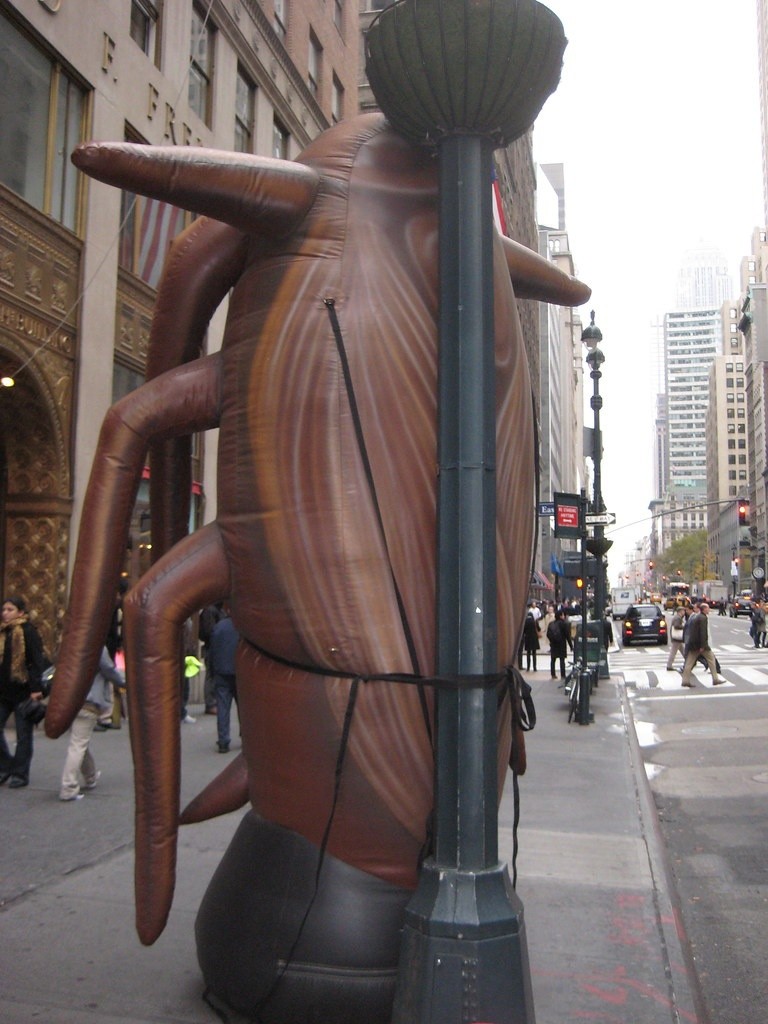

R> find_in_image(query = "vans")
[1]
[741,588,755,600]
[650,592,662,604]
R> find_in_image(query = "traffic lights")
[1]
[677,569,681,575]
[581,538,595,555]
[662,575,666,580]
[649,559,653,570]
[576,578,583,587]
[739,501,750,526]
[602,539,613,556]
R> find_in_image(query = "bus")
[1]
[663,581,690,597]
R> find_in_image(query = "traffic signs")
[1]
[585,513,616,526]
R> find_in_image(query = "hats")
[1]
[677,606,684,611]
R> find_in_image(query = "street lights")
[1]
[579,308,606,621]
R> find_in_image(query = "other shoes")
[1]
[85,770,101,788]
[183,716,197,724]
[682,683,695,687]
[713,680,726,685]
[60,793,84,801]
[10,776,26,787]
[205,706,217,714]
[667,668,676,670]
[0,770,10,784]
[219,745,229,752]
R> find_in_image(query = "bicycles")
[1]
[563,655,583,724]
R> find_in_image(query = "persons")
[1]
[528,596,594,637]
[53,629,125,800]
[201,600,225,713]
[0,596,43,788]
[639,598,642,603]
[547,611,574,678]
[210,604,237,752]
[682,595,691,606]
[672,598,679,616]
[603,615,613,650]
[524,612,541,671]
[750,601,768,648]
[181,618,196,722]
[681,603,727,687]
[98,580,129,729]
[718,597,727,616]
[666,603,721,673]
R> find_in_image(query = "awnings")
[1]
[530,572,553,590]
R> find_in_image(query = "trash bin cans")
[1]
[576,622,611,679]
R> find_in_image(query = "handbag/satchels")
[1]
[20,699,47,724]
[672,625,683,642]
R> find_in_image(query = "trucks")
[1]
[697,579,729,608]
[612,588,639,621]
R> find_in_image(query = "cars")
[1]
[646,591,653,599]
[729,597,754,618]
[622,604,669,648]
[663,596,689,611]
